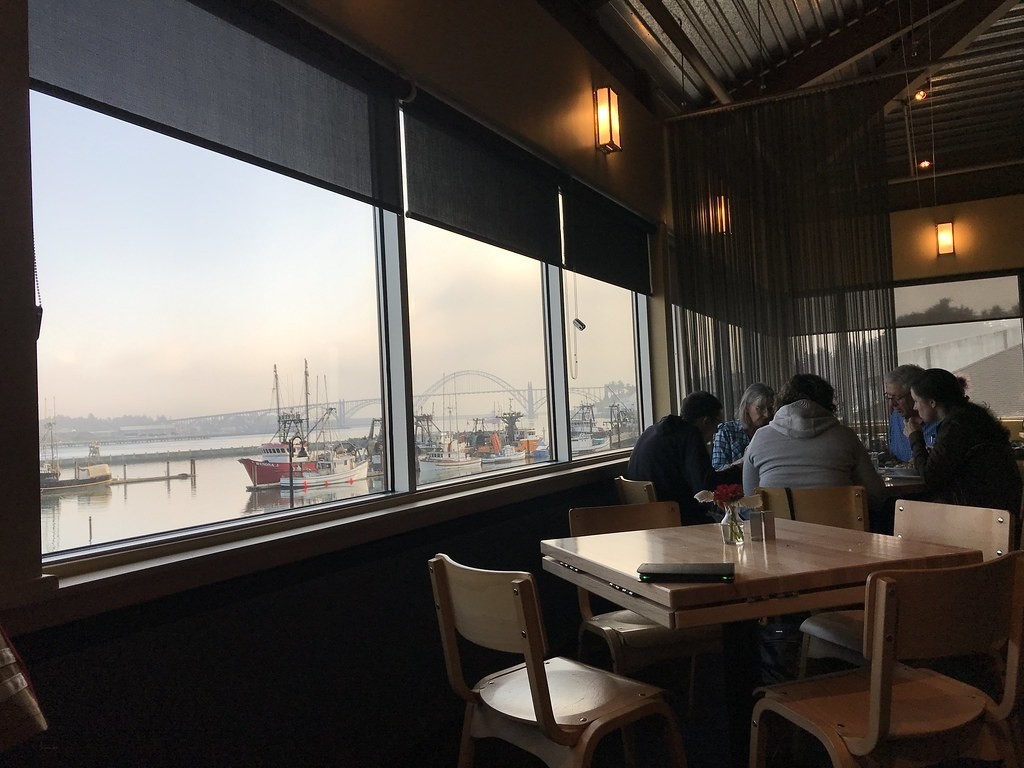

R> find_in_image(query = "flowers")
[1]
[694,483,744,545]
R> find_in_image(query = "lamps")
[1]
[917,159,931,169]
[914,90,927,101]
[591,83,623,156]
[935,222,955,255]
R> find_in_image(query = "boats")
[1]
[38,394,119,495]
[237,356,638,494]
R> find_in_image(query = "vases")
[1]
[720,506,744,546]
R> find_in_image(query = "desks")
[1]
[540,516,983,768]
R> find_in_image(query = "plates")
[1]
[884,475,919,478]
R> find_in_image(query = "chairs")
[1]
[569,501,723,768]
[753,486,870,531]
[1001,415,1024,520]
[427,552,688,768]
[613,475,657,504]
[749,550,1024,768]
[790,499,1024,768]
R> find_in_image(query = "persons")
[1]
[712,382,777,489]
[743,373,883,510]
[883,365,940,466]
[628,391,725,527]
[903,369,1024,550]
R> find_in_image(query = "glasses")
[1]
[751,404,777,416]
[883,392,910,400]
[702,417,725,429]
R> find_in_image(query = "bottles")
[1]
[871,452,878,471]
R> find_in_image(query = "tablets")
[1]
[637,562,736,584]
